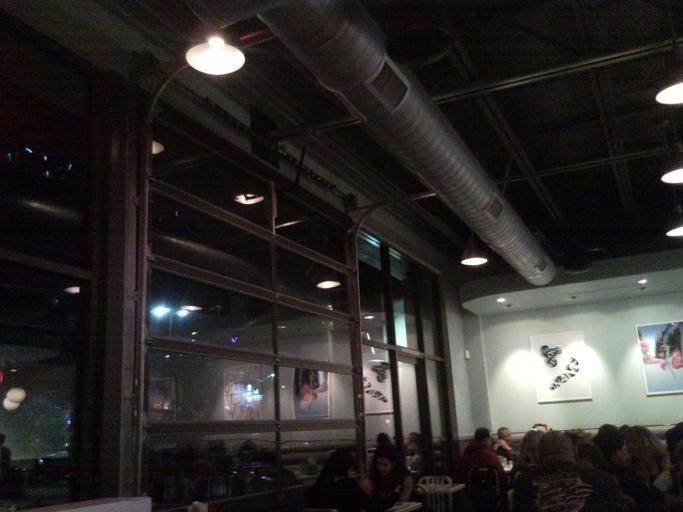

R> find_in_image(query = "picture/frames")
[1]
[529,331,593,405]
[358,359,394,416]
[221,361,263,422]
[634,319,683,398]
[291,356,332,421]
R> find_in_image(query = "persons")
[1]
[141,437,306,510]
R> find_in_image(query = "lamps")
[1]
[183,22,246,78]
[459,223,491,267]
